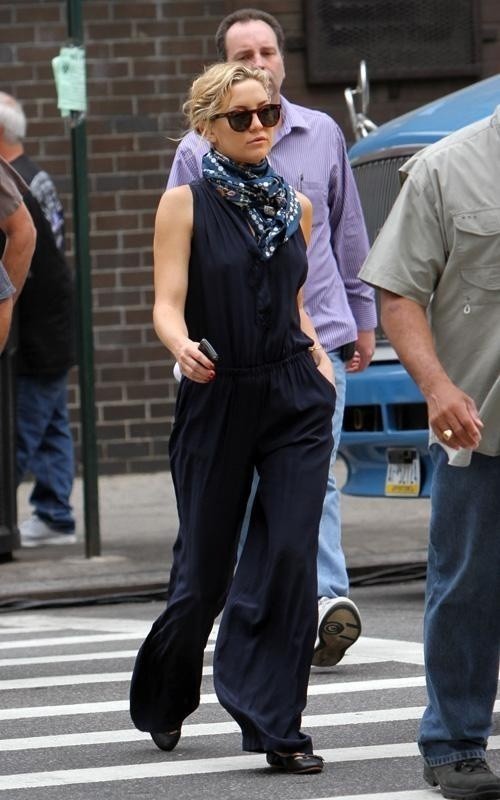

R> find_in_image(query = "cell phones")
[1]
[197,338,220,363]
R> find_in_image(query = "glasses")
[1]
[206,104,281,132]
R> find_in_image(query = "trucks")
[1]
[336,60,500,498]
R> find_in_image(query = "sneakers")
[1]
[17,515,77,548]
[311,596,362,667]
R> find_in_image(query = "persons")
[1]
[165,8,377,665]
[131,60,338,775]
[357,102,500,798]
[0,91,80,549]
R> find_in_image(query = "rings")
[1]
[442,429,453,441]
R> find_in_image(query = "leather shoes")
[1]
[0,552,12,563]
[149,731,181,751]
[422,758,499,800]
[266,750,325,774]
